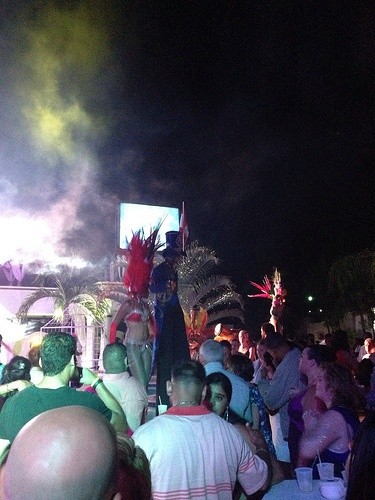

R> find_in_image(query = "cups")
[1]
[158,405,168,415]
[295,467,312,492]
[316,463,334,480]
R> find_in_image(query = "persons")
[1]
[109,279,156,398]
[150,247,194,406]
[0,321,375,499]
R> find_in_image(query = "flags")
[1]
[178,212,191,244]
